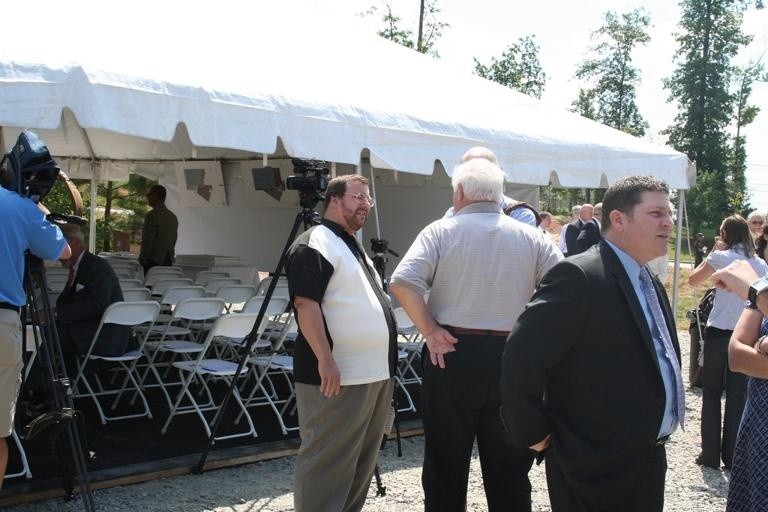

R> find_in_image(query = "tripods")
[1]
[191,208,388,497]
[22,251,97,512]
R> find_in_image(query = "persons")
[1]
[499,173,687,511]
[25,222,132,400]
[389,157,562,511]
[439,145,535,225]
[283,172,398,511]
[137,184,177,276]
[0,167,71,492]
[688,212,767,511]
[539,204,604,257]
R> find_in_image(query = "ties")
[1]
[640,268,685,432]
[67,268,74,284]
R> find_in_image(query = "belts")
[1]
[448,326,509,337]
[655,435,671,446]
[0,302,20,312]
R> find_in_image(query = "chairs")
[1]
[0,324,42,480]
[389,295,429,415]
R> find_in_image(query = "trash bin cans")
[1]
[687,309,727,389]
[113,227,130,251]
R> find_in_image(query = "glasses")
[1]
[345,192,376,207]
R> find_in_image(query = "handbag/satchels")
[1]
[693,288,715,320]
